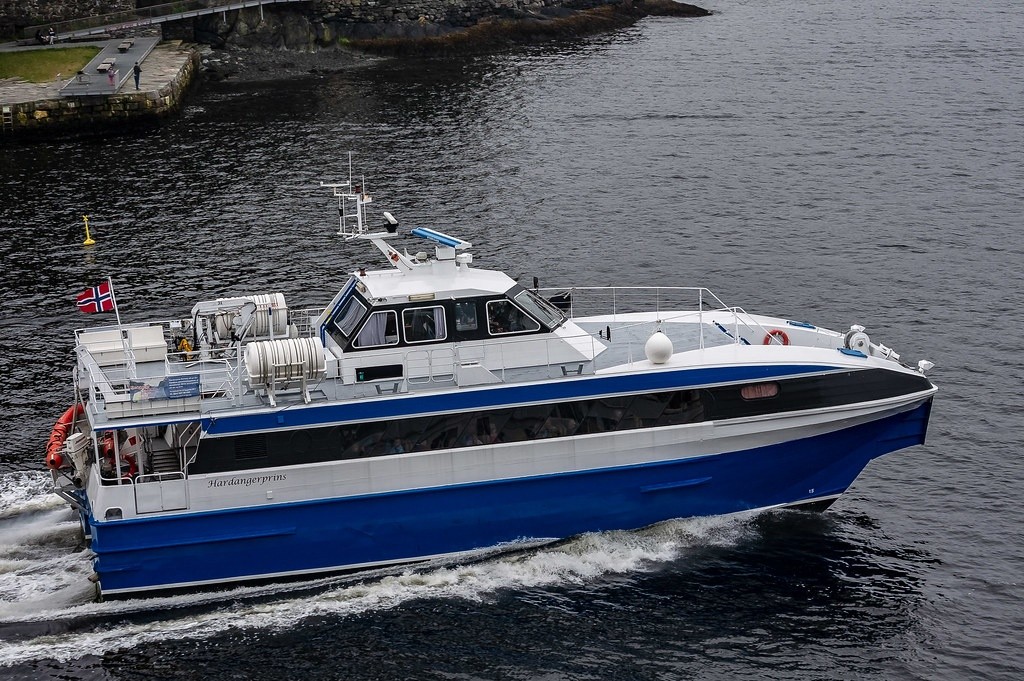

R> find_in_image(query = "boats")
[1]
[45,151,938,603]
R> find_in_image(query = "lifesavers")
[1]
[764,330,788,345]
[108,454,135,480]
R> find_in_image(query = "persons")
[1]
[489,301,512,334]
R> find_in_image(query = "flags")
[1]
[72,280,115,314]
